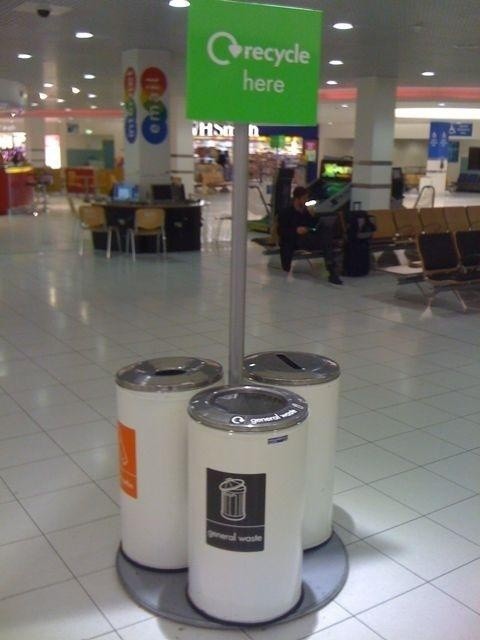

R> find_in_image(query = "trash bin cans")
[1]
[186,385,309,627]
[243,353,341,553]
[114,358,224,573]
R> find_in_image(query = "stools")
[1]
[26,181,49,216]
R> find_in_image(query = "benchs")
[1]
[206,173,227,186]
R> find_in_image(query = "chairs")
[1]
[367,210,415,240]
[420,208,449,232]
[444,207,472,232]
[467,206,480,230]
[416,232,480,313]
[79,206,121,258]
[127,208,167,261]
[393,208,421,238]
[454,231,480,272]
[68,198,79,241]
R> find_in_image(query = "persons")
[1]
[277,187,344,285]
[9,152,25,167]
[216,150,225,167]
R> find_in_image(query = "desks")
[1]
[92,201,211,253]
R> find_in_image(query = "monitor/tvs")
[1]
[152,184,172,204]
[113,183,138,203]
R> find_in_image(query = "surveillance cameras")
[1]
[37,7,51,17]
